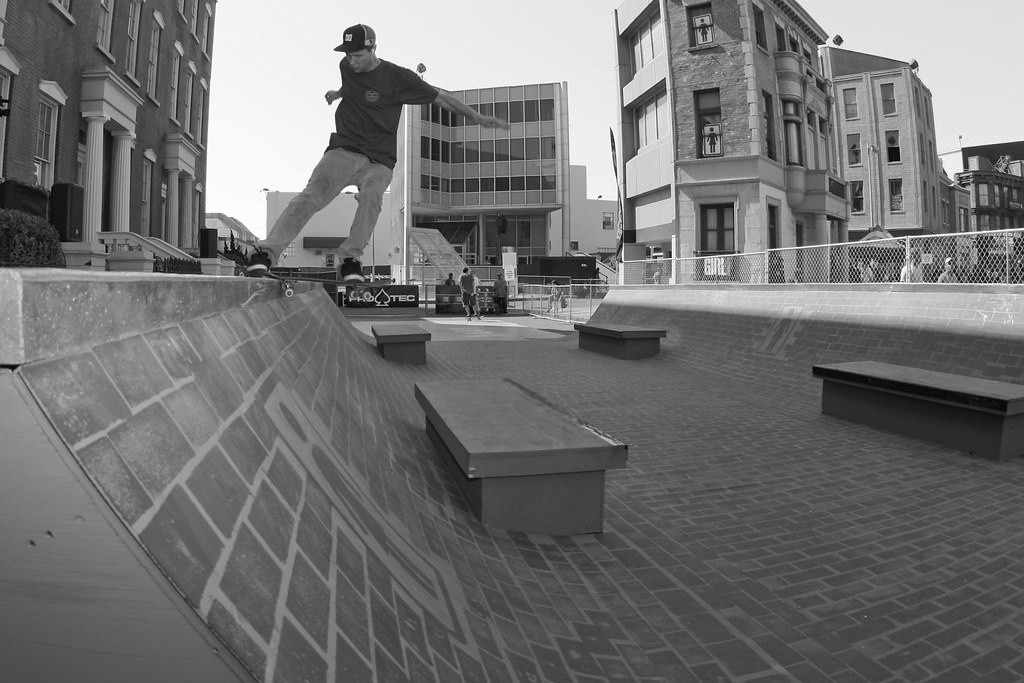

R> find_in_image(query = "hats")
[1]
[333,23,375,53]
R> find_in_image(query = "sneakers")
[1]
[340,257,365,283]
[246,245,268,272]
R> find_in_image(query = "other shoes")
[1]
[467,315,470,317]
[474,314,477,317]
[545,311,550,313]
[505,313,507,316]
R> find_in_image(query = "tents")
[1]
[849,226,905,248]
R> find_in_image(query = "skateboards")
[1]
[466,314,487,322]
[240,263,397,298]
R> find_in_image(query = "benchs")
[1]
[812,361,1024,462]
[574,324,667,360]
[371,324,431,365]
[414,377,627,534]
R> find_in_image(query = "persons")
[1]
[858,258,885,282]
[446,273,455,285]
[544,280,567,313]
[494,272,508,315]
[900,248,923,282]
[938,257,958,283]
[458,267,477,316]
[246,24,511,283]
[653,268,661,284]
[470,271,480,285]
